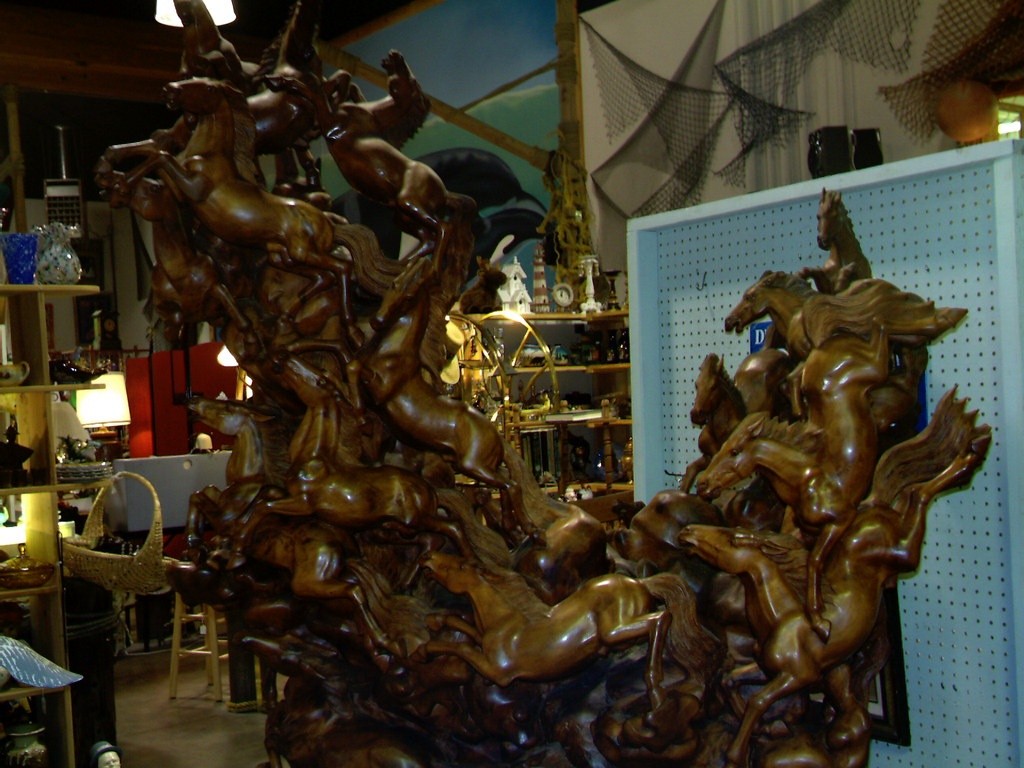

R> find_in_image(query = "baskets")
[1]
[62,469,179,595]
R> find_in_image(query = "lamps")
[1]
[74,370,131,461]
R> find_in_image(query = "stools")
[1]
[167,560,229,704]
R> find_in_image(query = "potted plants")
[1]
[54,434,113,482]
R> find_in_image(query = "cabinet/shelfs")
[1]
[0,283,113,768]
[482,305,634,532]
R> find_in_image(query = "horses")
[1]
[97,36,992,768]
